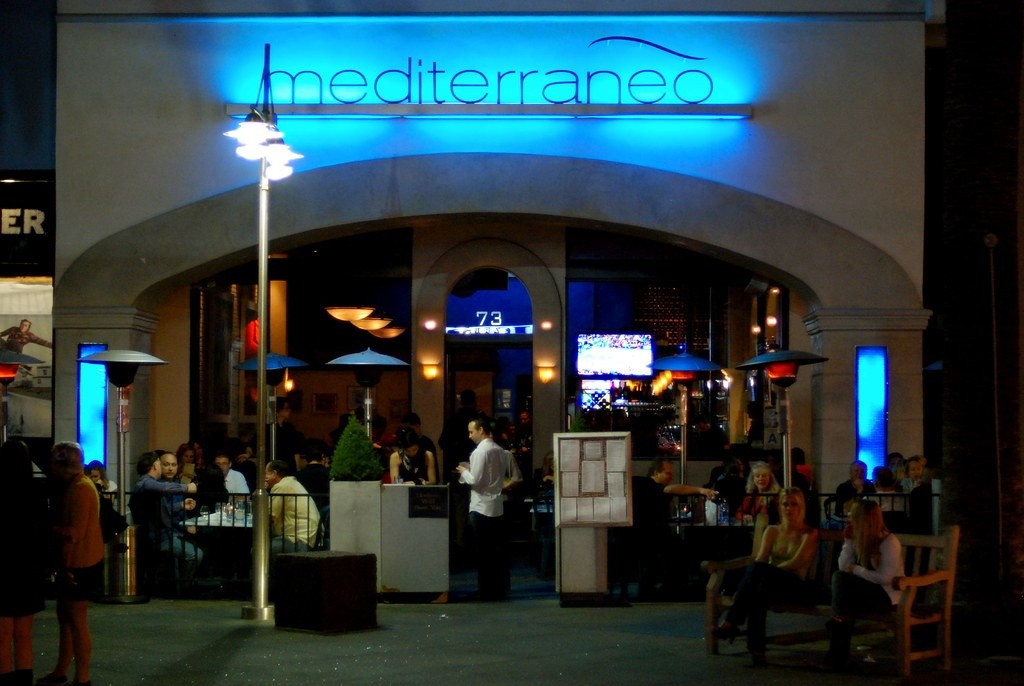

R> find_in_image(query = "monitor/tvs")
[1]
[573,328,657,395]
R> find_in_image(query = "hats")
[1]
[50,441,84,473]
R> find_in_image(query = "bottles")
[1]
[720,499,730,524]
[610,381,653,403]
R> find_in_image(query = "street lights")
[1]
[222,45,305,624]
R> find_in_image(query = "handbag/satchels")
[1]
[97,491,129,541]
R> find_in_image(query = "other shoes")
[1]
[820,652,851,671]
[66,680,92,686]
[36,672,67,683]
[825,615,848,628]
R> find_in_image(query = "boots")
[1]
[0,671,16,686]
[15,669,34,686]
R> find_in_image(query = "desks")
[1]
[668,523,756,578]
[179,501,255,598]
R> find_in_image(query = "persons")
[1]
[478,409,532,482]
[0,438,45,686]
[808,500,906,674]
[127,397,439,599]
[84,461,117,503]
[456,412,524,602]
[0,319,52,354]
[534,452,554,582]
[35,442,105,686]
[711,487,817,668]
[587,406,995,593]
[437,390,479,548]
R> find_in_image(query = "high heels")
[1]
[712,625,740,644]
[743,652,769,669]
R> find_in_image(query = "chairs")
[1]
[534,497,555,578]
[311,505,332,552]
[825,497,845,529]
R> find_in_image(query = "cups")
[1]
[394,475,404,482]
[200,502,244,523]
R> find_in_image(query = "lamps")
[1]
[325,234,406,339]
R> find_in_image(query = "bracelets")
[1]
[700,489,705,495]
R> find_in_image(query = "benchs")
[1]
[705,523,960,680]
